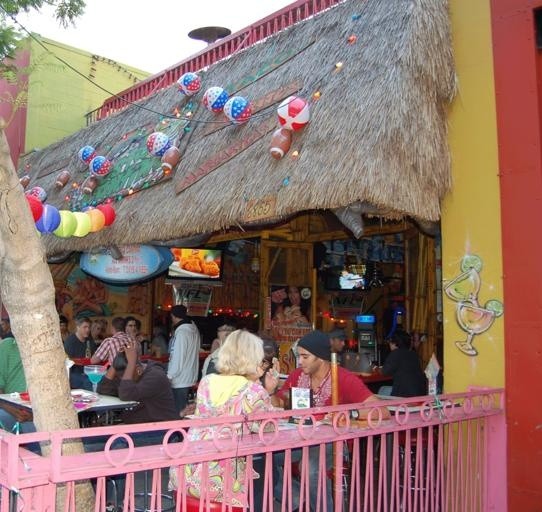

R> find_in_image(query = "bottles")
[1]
[251,241,262,274]
[85,341,92,358]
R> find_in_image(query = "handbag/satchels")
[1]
[343,352,372,372]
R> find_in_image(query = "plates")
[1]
[169,261,219,278]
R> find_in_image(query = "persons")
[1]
[0,314,176,512]
[261,340,276,371]
[328,329,346,353]
[211,324,236,352]
[264,328,392,512]
[167,327,279,512]
[275,286,312,325]
[167,304,201,419]
[377,330,426,407]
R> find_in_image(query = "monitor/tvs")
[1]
[164,245,224,281]
[322,263,370,293]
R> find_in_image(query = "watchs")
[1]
[350,409,359,419]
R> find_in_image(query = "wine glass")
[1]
[84,365,108,397]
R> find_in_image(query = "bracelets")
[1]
[268,392,276,396]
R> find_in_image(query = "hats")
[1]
[297,329,331,360]
[171,304,187,317]
[329,329,348,340]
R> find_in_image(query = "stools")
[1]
[292,431,437,512]
[82,429,243,512]
